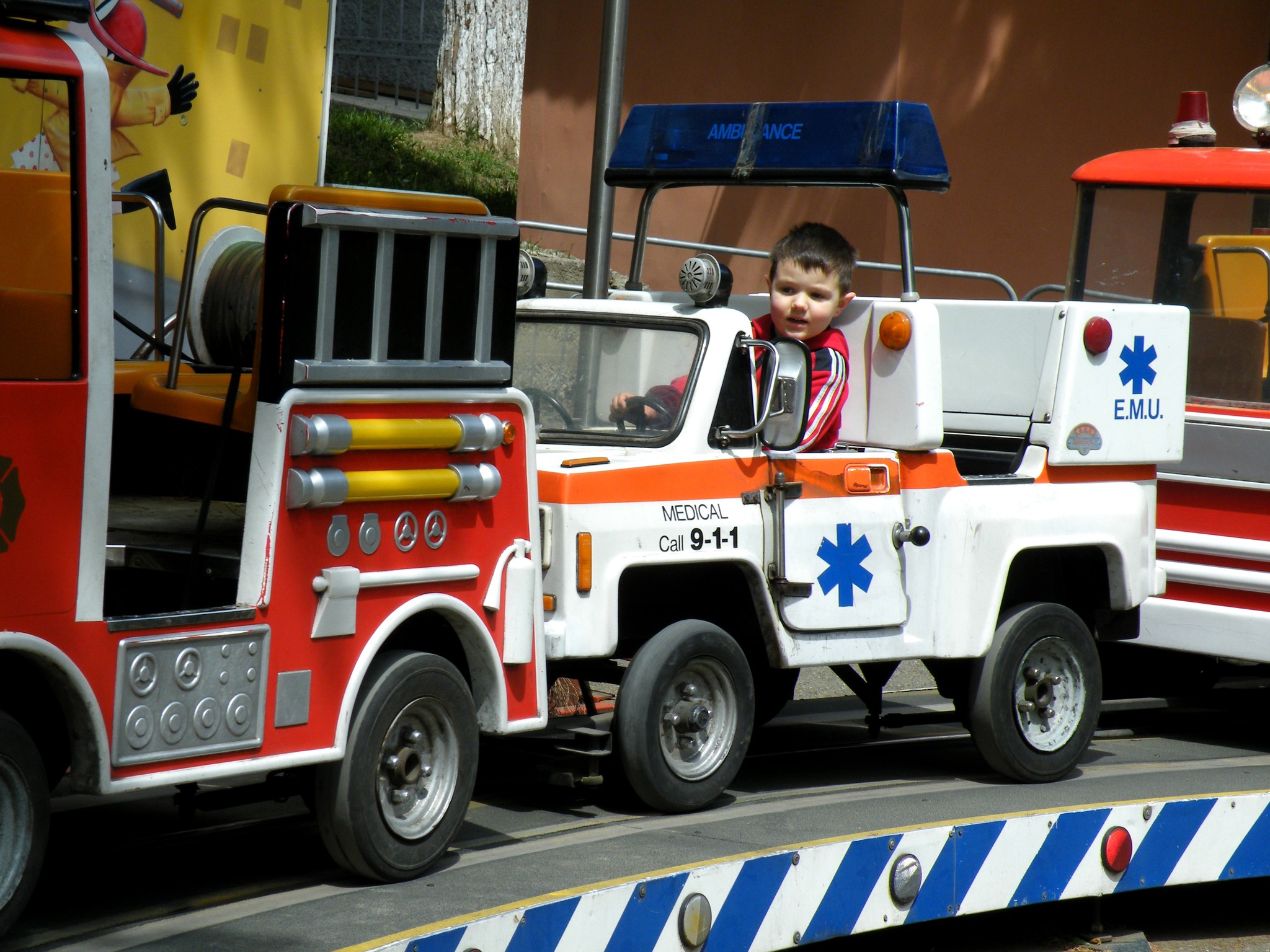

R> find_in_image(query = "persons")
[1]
[609,218,861,457]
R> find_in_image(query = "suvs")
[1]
[0,0,1270,952]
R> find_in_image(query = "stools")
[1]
[114,340,268,484]
